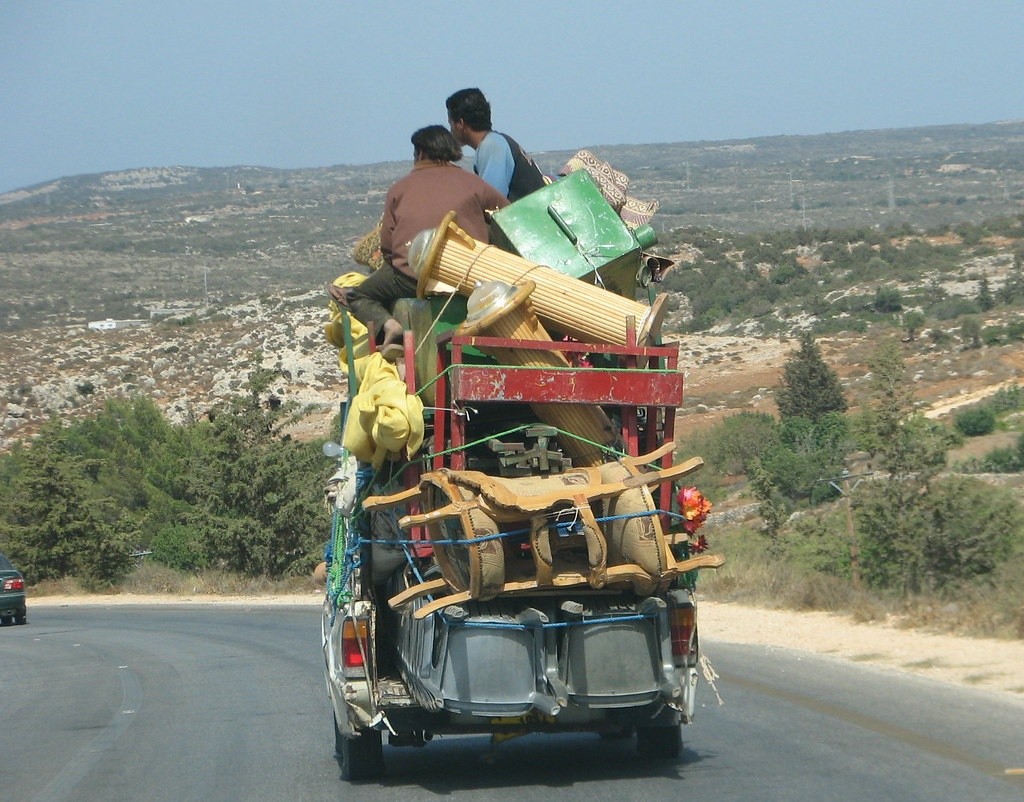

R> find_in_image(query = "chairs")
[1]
[385,590,691,723]
[358,442,726,621]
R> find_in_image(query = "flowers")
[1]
[677,485,712,555]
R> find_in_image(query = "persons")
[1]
[442,86,545,209]
[345,124,513,351]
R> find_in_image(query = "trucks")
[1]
[320,403,697,782]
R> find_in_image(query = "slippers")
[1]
[327,283,350,311]
[377,343,402,360]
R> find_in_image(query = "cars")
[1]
[0,554,27,625]
